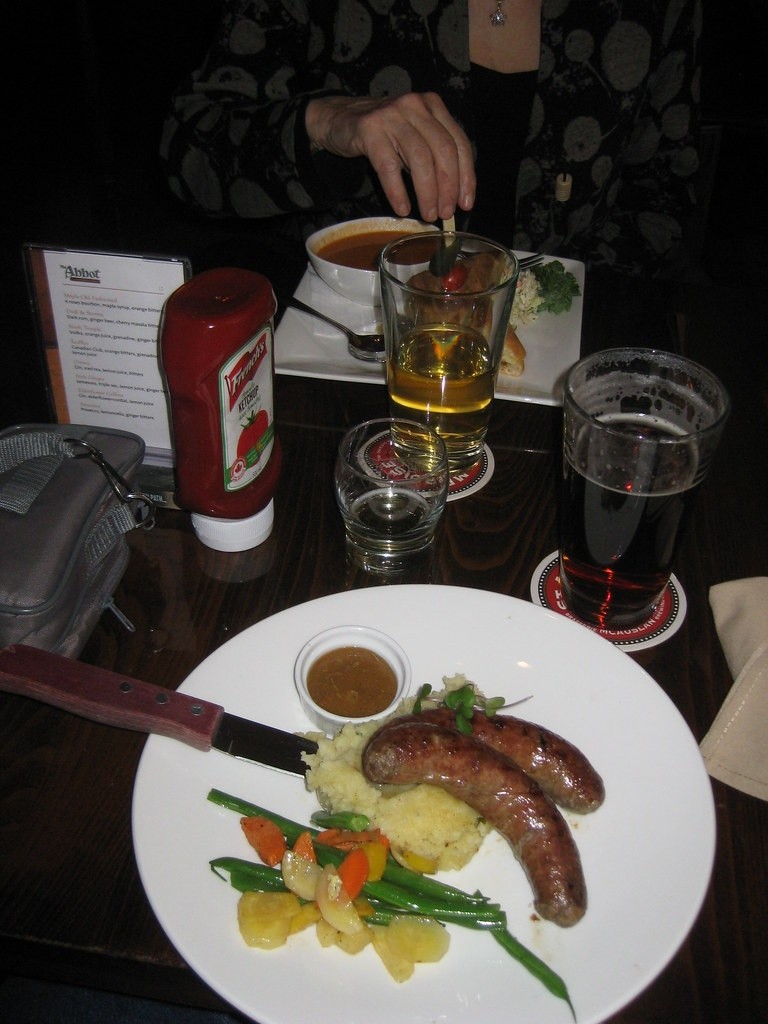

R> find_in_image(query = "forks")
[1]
[458,251,544,270]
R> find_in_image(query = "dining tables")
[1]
[0,227,768,1024]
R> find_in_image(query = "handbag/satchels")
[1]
[0,423,167,662]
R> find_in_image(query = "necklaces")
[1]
[488,1,507,26]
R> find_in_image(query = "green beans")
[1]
[207,789,577,1024]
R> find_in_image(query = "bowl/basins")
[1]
[305,216,442,307]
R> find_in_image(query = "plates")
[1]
[273,248,585,406]
[133,585,718,1024]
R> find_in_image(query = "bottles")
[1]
[156,266,283,552]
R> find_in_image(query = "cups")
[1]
[295,624,411,736]
[380,231,519,471]
[559,347,731,629]
[335,416,449,552]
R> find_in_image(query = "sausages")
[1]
[363,722,588,928]
[362,706,605,815]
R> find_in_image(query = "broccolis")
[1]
[528,258,581,316]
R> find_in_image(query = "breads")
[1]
[403,249,526,378]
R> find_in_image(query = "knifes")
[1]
[0,644,319,772]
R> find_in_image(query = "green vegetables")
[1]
[411,683,534,738]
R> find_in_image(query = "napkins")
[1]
[697,576,768,802]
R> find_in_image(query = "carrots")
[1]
[238,816,287,868]
[293,831,317,866]
[335,848,369,902]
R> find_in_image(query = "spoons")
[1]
[288,297,386,362]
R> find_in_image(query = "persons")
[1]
[148,1,714,326]
